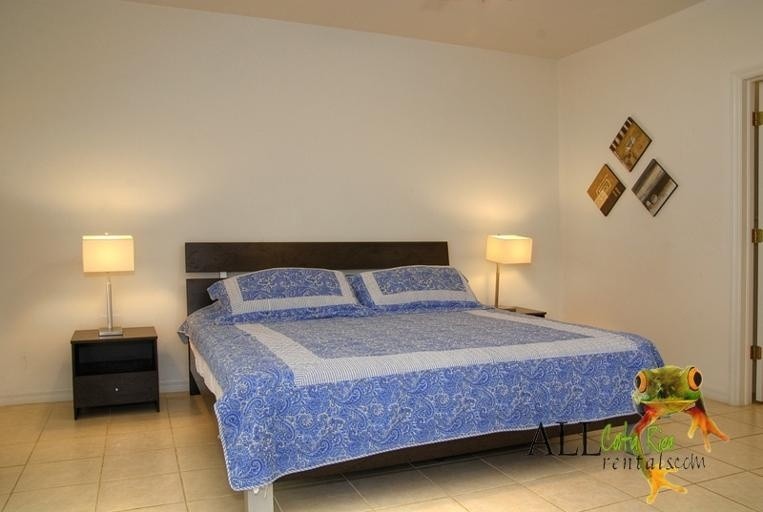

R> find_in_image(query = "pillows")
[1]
[207,264,494,326]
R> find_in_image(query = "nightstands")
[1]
[70,327,160,421]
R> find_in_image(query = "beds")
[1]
[185,242,663,511]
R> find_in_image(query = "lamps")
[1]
[485,234,533,311]
[82,232,135,336]
